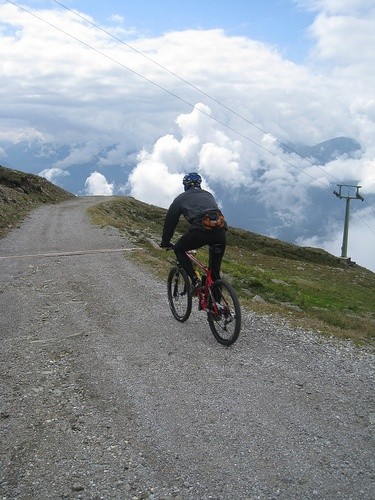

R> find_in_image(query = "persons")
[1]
[161,172,228,321]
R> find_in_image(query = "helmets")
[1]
[183,173,202,184]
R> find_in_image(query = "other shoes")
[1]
[188,278,203,297]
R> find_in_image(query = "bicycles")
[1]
[159,242,241,346]
